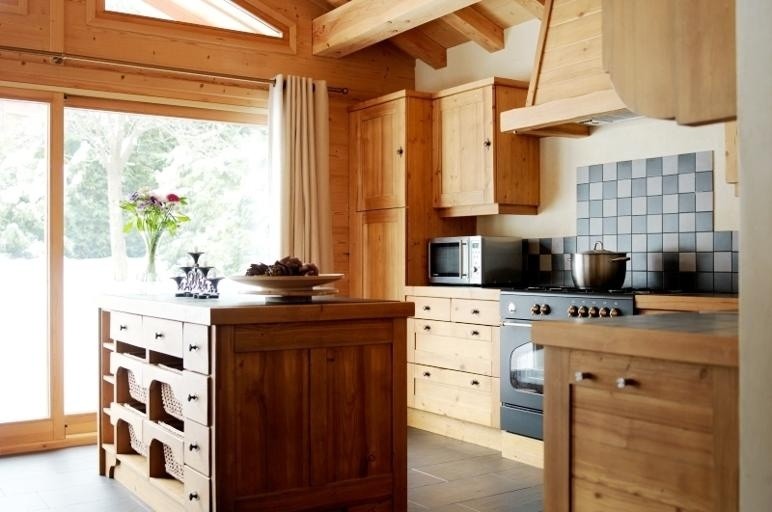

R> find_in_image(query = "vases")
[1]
[139,231,163,283]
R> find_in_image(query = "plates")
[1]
[228,273,347,297]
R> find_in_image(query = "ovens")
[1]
[498,319,546,442]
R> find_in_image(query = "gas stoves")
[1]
[499,286,684,319]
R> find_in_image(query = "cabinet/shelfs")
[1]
[405,285,500,450]
[347,88,477,301]
[98,308,407,512]
[433,76,541,217]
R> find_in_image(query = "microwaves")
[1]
[425,235,522,288]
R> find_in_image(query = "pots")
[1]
[570,241,630,290]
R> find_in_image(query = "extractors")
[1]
[500,0,643,137]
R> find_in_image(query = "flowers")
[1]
[117,187,192,236]
